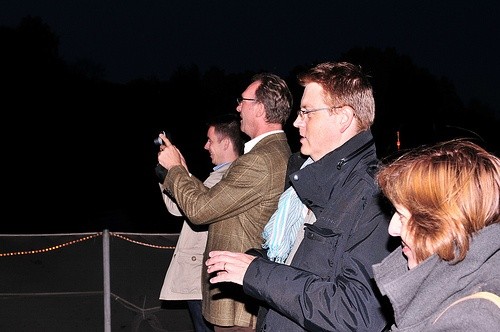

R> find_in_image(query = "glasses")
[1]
[297,107,338,119]
[236,97,258,104]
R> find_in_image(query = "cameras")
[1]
[153,131,170,145]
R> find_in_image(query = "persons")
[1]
[159,113,251,332]
[203,59,404,331]
[372,138,499,332]
[156,72,299,330]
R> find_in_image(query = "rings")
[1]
[224,263,227,270]
